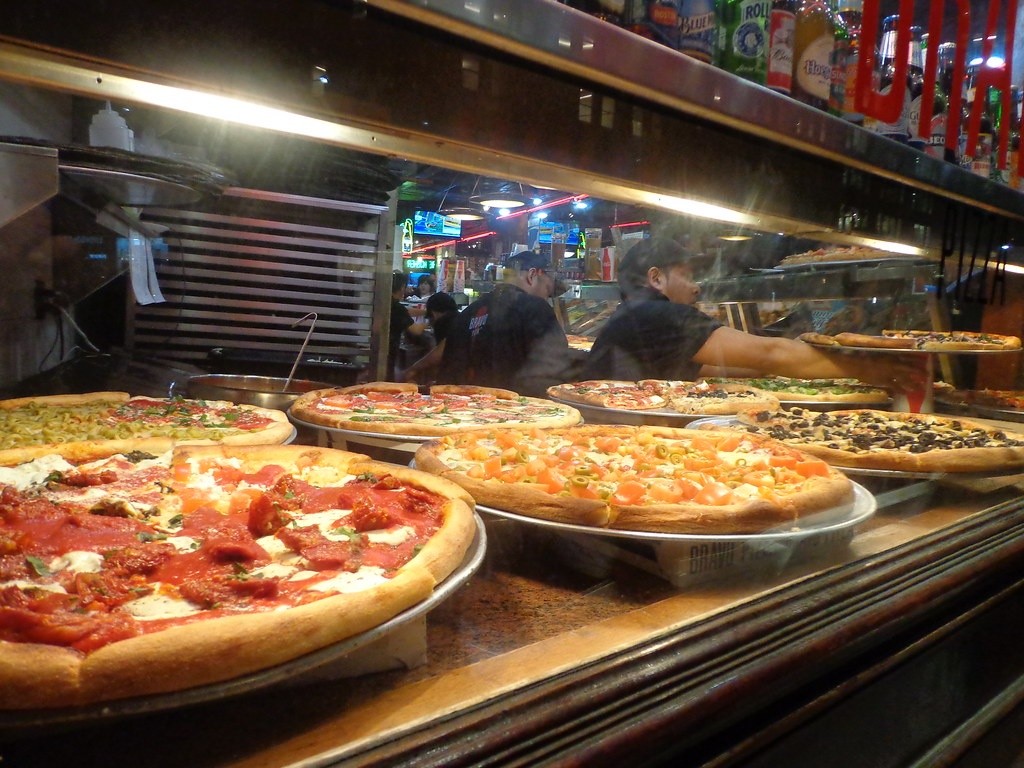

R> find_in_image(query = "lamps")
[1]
[435,172,494,221]
[469,176,532,208]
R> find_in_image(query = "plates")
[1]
[0,494,488,731]
[783,257,927,268]
[933,394,1024,423]
[680,413,953,483]
[548,391,739,431]
[410,454,880,548]
[775,397,898,414]
[805,340,1024,356]
[285,406,459,445]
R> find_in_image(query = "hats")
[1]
[424,291,458,318]
[618,237,717,278]
[504,250,572,298]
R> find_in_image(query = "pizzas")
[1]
[416,426,852,535]
[1,393,294,464]
[778,248,901,265]
[700,410,1024,473]
[547,379,779,415]
[0,445,476,707]
[291,382,579,436]
[800,330,1021,351]
[956,389,1024,422]
[697,376,888,401]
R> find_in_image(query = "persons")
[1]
[405,274,437,298]
[386,274,430,382]
[580,236,930,392]
[439,251,572,398]
[403,292,461,385]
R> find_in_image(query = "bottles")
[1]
[562,0,1024,195]
[602,249,611,280]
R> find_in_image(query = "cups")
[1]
[437,259,450,293]
[453,259,465,293]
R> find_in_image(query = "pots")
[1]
[169,373,340,444]
